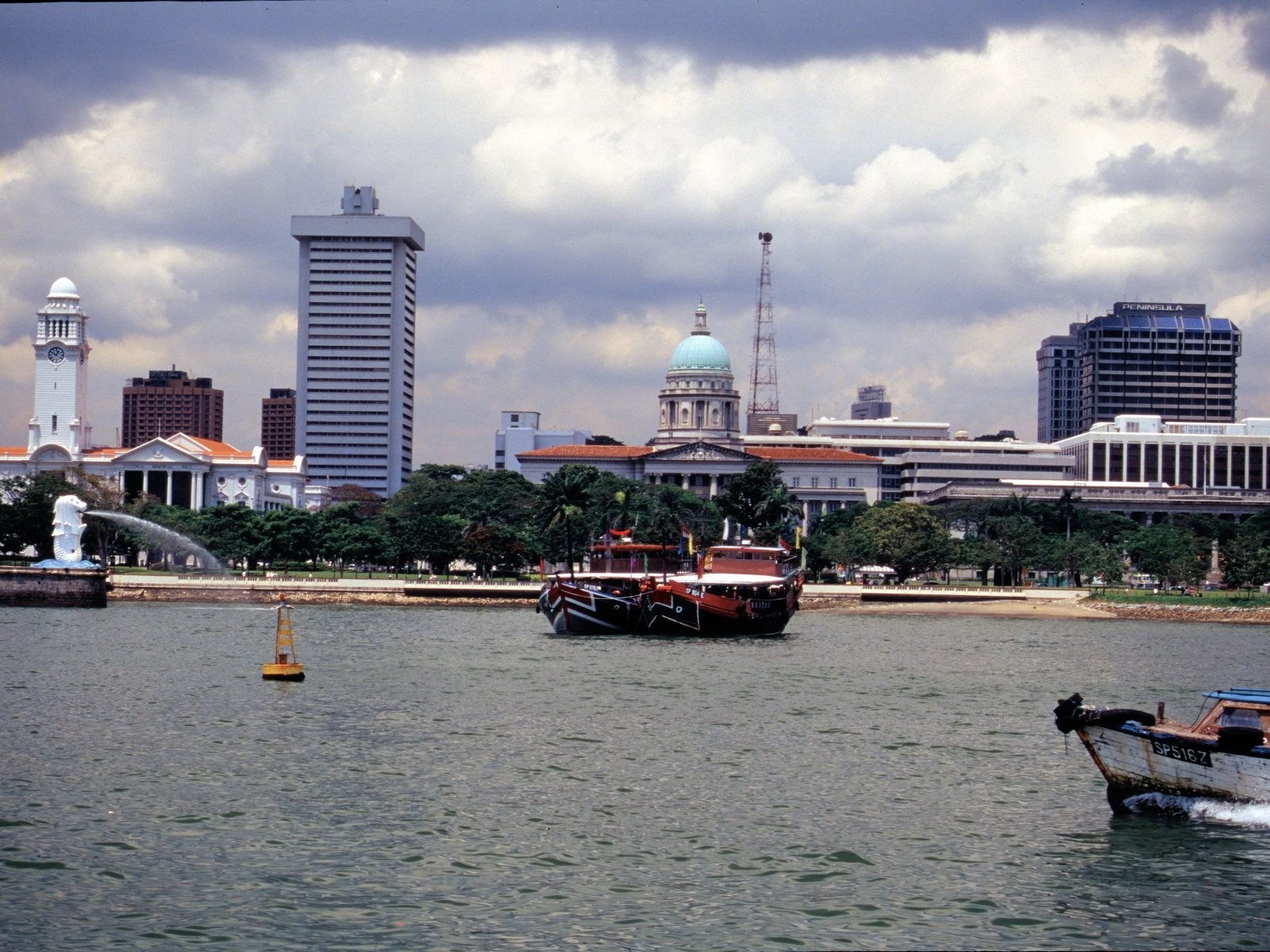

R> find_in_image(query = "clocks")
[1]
[48,346,65,363]
[80,349,85,364]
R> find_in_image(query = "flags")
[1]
[778,540,792,556]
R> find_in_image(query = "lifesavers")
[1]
[1053,693,1083,718]
[1217,727,1264,748]
[536,605,541,613]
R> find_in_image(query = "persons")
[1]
[1181,587,1194,596]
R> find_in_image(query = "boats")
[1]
[534,510,806,639]
[1051,688,1270,821]
